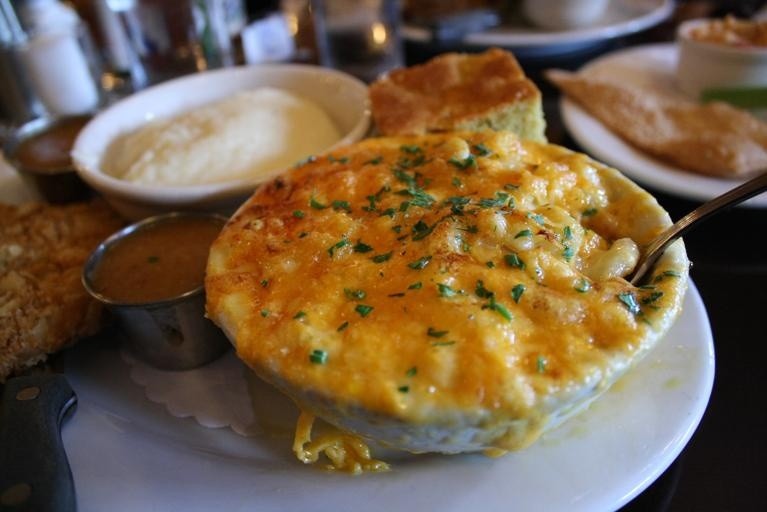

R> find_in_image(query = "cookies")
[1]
[0,198,126,380]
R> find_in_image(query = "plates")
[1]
[64,278,720,512]
[395,1,680,58]
[559,38,765,213]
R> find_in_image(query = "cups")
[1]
[13,28,100,114]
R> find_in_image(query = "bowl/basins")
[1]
[69,64,370,221]
[8,92,117,207]
[81,209,231,366]
[678,16,766,104]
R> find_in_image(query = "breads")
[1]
[369,46,548,143]
